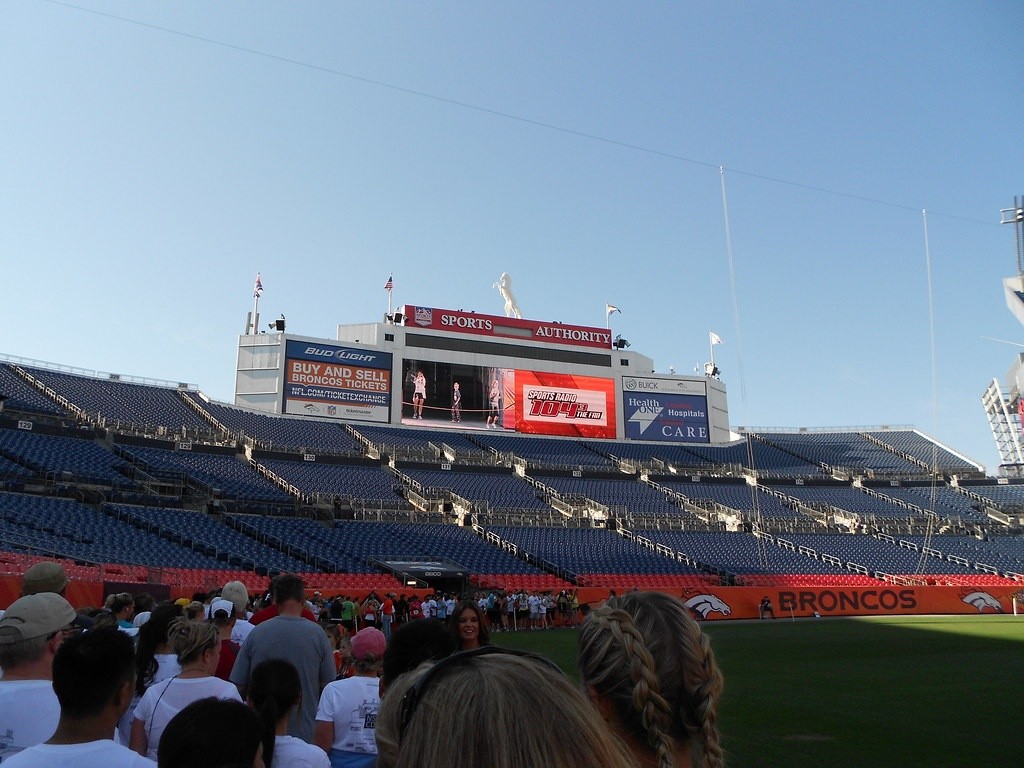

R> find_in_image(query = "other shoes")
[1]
[492,423,497,428]
[486,423,490,429]
[457,419,462,423]
[413,414,416,419]
[418,416,422,420]
[449,419,456,423]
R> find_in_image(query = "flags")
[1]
[253,275,264,300]
[384,276,394,292]
[710,332,723,345]
[608,305,622,316]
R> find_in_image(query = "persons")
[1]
[157,696,266,767]
[247,659,331,767]
[315,627,387,767]
[21,562,396,762]
[609,590,616,598]
[486,380,499,428]
[1,628,158,768]
[0,592,95,762]
[760,595,776,619]
[396,589,579,634]
[450,382,462,423]
[374,601,641,767]
[578,592,724,767]
[411,370,426,419]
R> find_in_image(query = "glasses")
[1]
[398,646,566,751]
[47,623,84,641]
[378,663,384,675]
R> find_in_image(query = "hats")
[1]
[208,600,236,619]
[351,626,387,662]
[175,598,191,610]
[22,561,67,595]
[133,612,151,627]
[0,592,95,644]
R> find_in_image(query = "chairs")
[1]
[0,361,1024,591]
